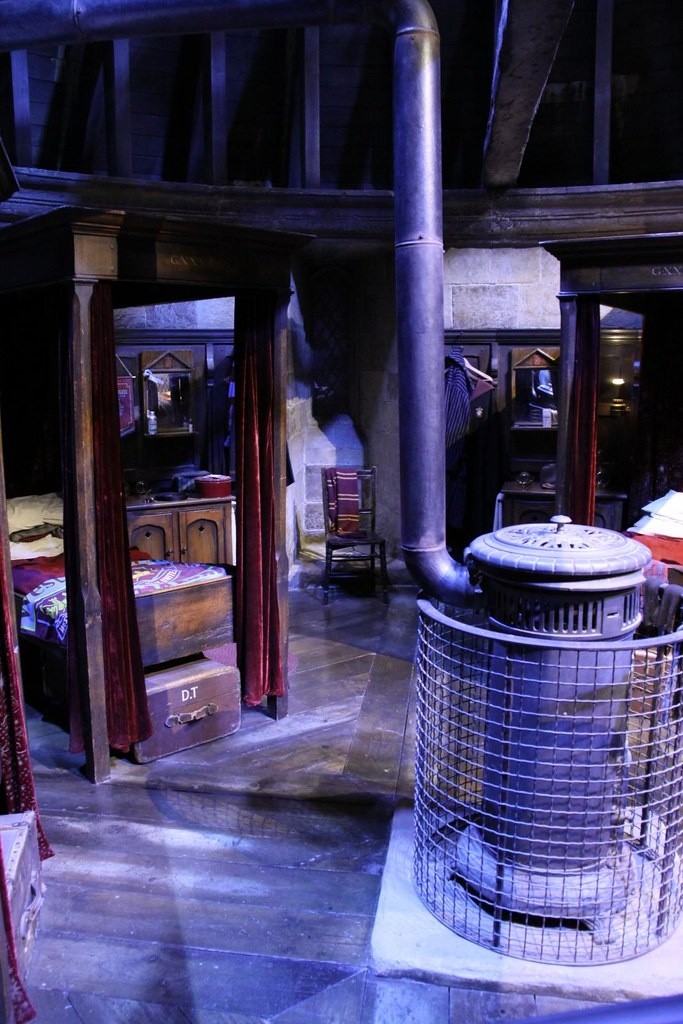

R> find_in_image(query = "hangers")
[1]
[444,342,494,382]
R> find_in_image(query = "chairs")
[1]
[321,466,389,606]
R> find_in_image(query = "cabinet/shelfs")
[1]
[126,501,233,566]
[114,329,236,495]
[445,330,563,564]
[501,481,627,532]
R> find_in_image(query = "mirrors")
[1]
[141,349,201,440]
[511,346,561,430]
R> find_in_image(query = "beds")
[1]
[5,492,236,697]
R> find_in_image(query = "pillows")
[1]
[7,493,64,560]
[627,490,683,539]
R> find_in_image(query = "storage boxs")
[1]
[0,811,49,984]
[130,652,241,764]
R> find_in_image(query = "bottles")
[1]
[149,411,157,435]
